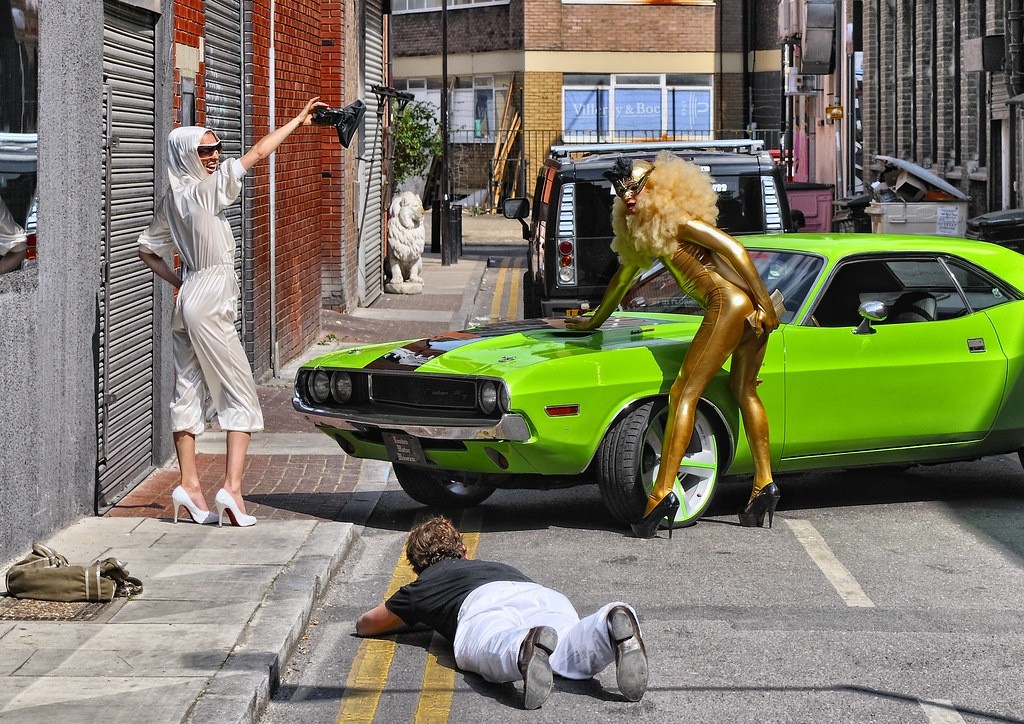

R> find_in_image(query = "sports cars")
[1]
[290,229,1024,532]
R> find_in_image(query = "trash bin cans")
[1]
[864,154,973,238]
[847,196,875,234]
[964,208,1024,255]
[784,181,835,233]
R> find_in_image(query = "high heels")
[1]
[738,482,780,528]
[172,485,219,524]
[216,488,258,527]
[630,491,680,539]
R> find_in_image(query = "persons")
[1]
[356,517,649,710]
[564,158,781,538]
[0,195,28,275]
[137,96,331,527]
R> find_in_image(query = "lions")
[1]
[383,190,427,285]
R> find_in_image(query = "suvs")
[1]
[502,135,809,326]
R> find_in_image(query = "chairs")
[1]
[856,290,938,325]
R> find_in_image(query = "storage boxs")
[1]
[895,172,927,202]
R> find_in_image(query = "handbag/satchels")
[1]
[6,542,143,602]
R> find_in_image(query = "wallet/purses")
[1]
[746,289,787,339]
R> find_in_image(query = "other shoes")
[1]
[518,626,558,710]
[608,606,649,702]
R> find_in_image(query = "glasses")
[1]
[198,140,223,159]
